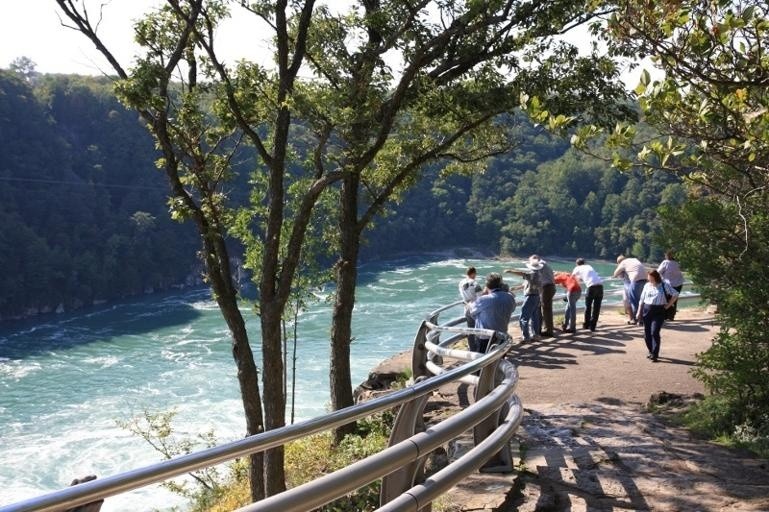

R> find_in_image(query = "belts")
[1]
[636,279,647,282]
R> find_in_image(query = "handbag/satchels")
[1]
[663,303,676,320]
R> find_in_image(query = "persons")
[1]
[660,252,687,320]
[467,271,516,363]
[528,253,557,338]
[612,254,649,326]
[572,259,605,331]
[634,266,679,360]
[503,260,545,344]
[552,268,582,333]
[458,266,483,305]
[618,271,636,324]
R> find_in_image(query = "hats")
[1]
[526,259,544,270]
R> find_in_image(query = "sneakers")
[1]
[522,331,553,344]
[628,320,643,326]
[647,353,658,361]
[561,322,576,333]
[583,321,595,332]
